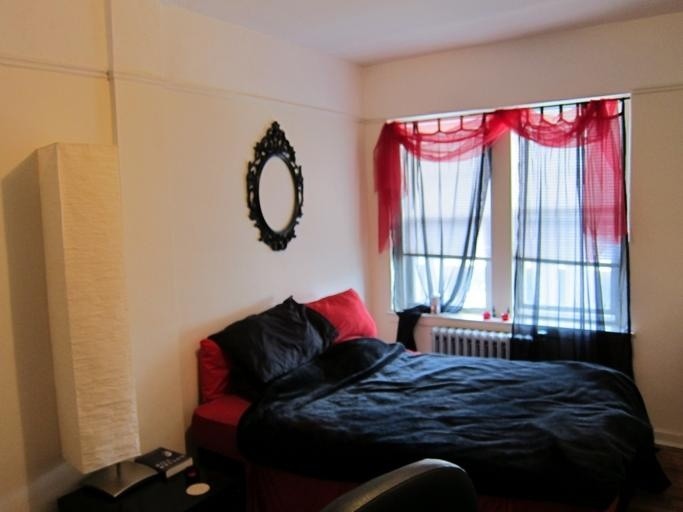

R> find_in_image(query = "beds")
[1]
[192,288,673,512]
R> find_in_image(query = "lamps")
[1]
[34,140,160,501]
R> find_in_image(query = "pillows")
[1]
[308,288,375,343]
[200,338,232,402]
[216,294,324,390]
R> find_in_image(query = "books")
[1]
[135,446,193,479]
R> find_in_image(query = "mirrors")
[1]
[246,122,304,251]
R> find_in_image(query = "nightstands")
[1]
[55,448,246,511]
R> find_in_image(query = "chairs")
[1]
[318,459,477,512]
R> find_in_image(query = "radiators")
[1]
[431,326,549,359]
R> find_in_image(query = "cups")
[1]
[501,312,508,320]
[483,311,492,320]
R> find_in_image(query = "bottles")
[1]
[430,294,441,315]
